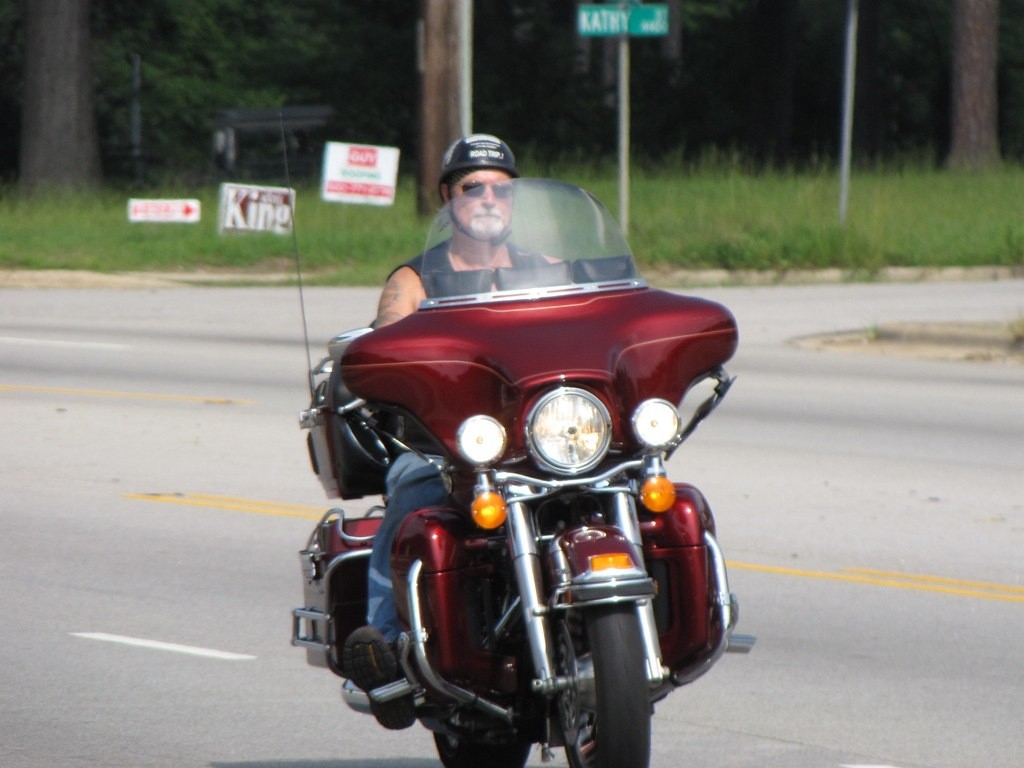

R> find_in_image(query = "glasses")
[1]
[457,180,518,199]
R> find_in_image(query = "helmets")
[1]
[440,133,518,178]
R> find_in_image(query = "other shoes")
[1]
[340,624,419,732]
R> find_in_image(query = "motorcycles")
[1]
[276,111,757,768]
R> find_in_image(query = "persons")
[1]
[340,133,583,731]
